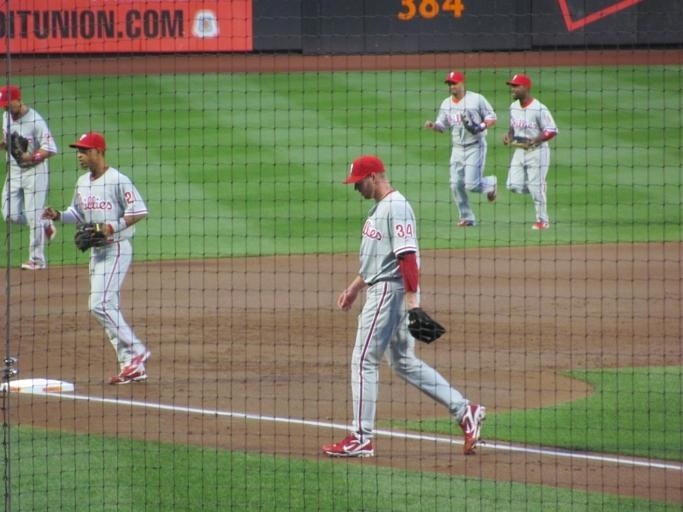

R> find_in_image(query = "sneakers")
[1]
[457,219,474,227]
[321,432,374,458]
[21,261,46,270]
[42,219,55,242]
[531,218,550,230]
[487,176,496,201]
[457,402,486,455]
[106,348,151,387]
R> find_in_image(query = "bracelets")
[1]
[479,122,487,131]
[31,149,42,164]
[108,217,127,235]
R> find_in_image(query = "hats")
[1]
[342,156,385,184]
[0,86,20,108]
[505,74,531,90]
[444,71,465,84]
[69,134,105,152]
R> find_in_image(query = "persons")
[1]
[39,131,152,386]
[503,74,559,231]
[0,84,57,271]
[424,71,497,228]
[320,157,487,460]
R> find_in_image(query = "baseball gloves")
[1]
[508,136,540,151]
[75,223,115,252]
[408,308,445,345]
[461,108,482,135]
[1,131,28,171]
